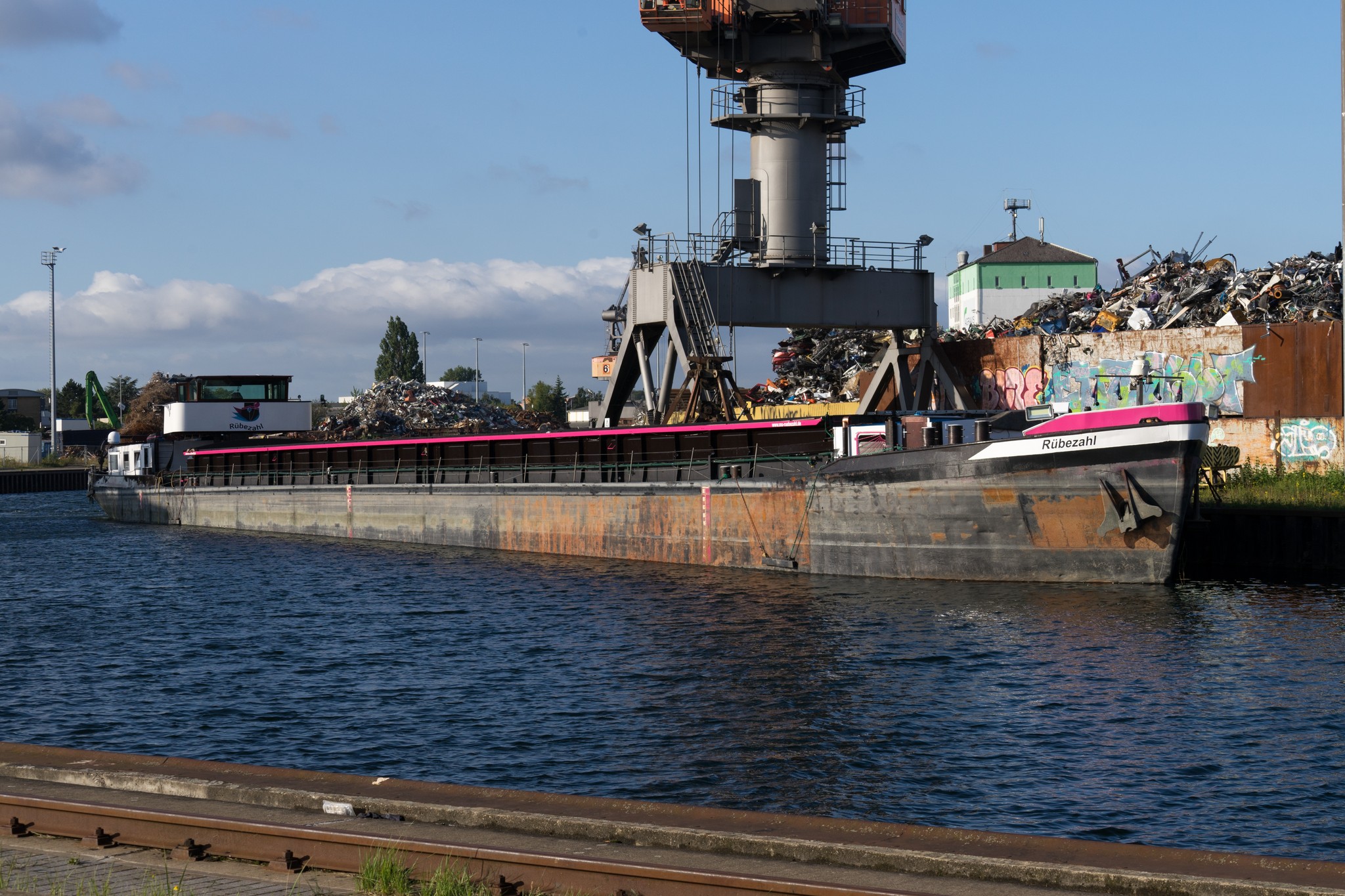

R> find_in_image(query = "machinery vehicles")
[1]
[85,369,123,431]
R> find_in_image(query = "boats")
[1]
[85,366,1215,591]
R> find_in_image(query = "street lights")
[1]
[520,343,530,410]
[473,338,482,403]
[118,374,123,424]
[420,331,431,383]
[41,245,68,454]
